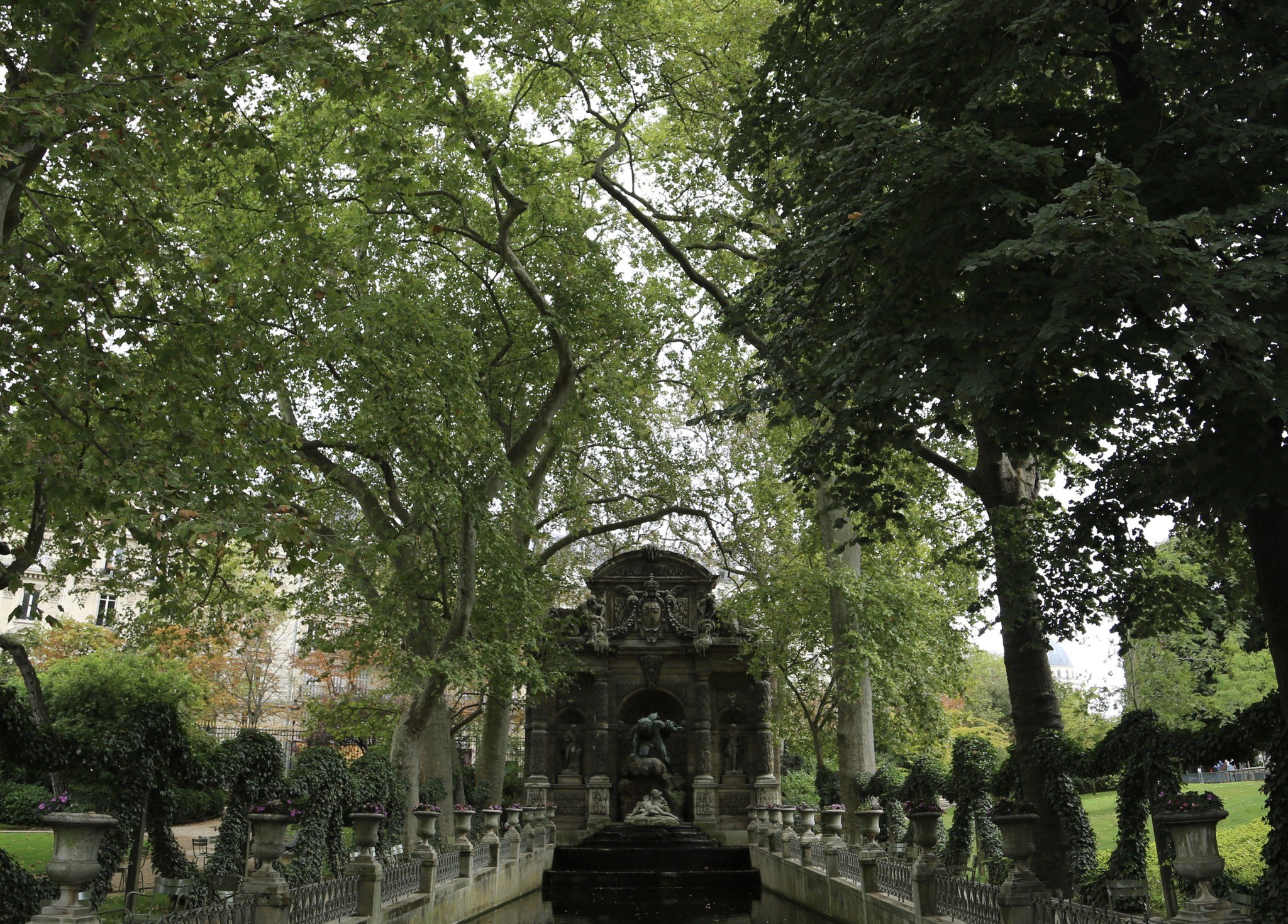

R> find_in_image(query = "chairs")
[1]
[192,836,219,870]
[333,844,411,878]
[116,845,151,893]
[282,824,301,859]
[840,833,920,866]
[948,853,979,882]
[441,832,481,855]
[1105,879,1149,921]
[1230,893,1256,924]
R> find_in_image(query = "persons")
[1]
[562,724,583,777]
[627,790,680,821]
[620,717,678,762]
[549,595,605,623]
[697,593,738,632]
[719,724,742,775]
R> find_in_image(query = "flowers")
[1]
[249,799,556,816]
[748,796,1038,816]
[1156,790,1227,814]
[35,790,96,816]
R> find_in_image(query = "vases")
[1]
[39,812,119,917]
[240,806,558,893]
[1152,806,1231,924]
[746,807,1045,894]
